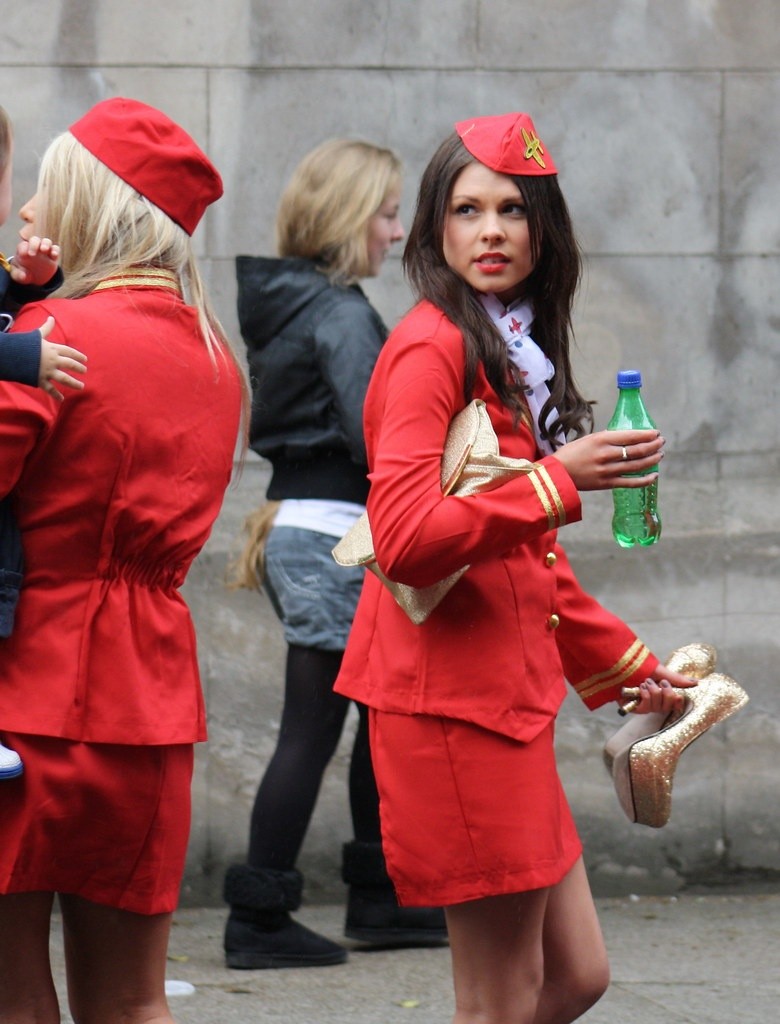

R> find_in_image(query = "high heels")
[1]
[612,672,751,828]
[604,643,717,776]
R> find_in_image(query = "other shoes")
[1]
[0,746,23,778]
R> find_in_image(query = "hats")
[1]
[69,98,224,238]
[454,113,558,176]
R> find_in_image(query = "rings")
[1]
[622,446,628,461]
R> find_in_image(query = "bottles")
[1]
[606,369,662,550]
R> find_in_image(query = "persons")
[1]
[224,137,452,966]
[330,114,698,1024]
[0,98,253,1024]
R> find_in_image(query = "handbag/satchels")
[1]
[332,400,534,625]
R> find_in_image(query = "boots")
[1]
[341,839,451,942]
[223,864,345,968]
